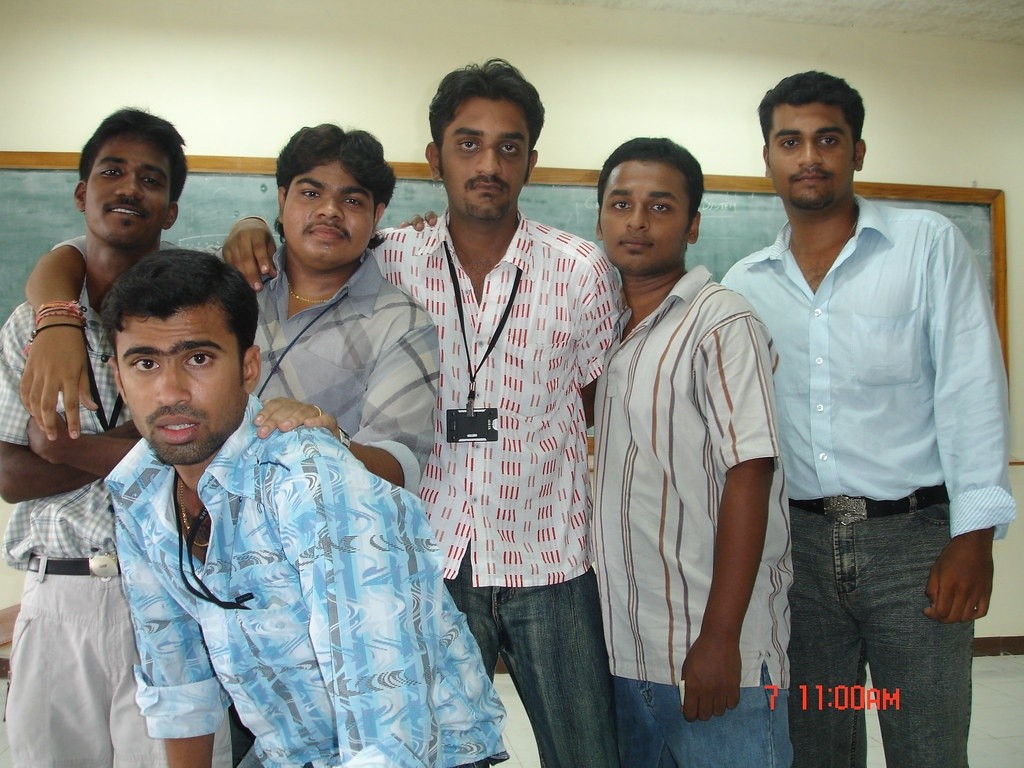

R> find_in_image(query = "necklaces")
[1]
[288,283,330,303]
[840,220,857,246]
[179,481,209,546]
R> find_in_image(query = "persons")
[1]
[24,123,438,768]
[222,59,627,767]
[400,137,796,767]
[720,71,1017,768]
[97,249,509,768]
[0,106,187,768]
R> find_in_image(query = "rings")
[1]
[313,405,323,417]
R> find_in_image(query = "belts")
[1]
[788,482,950,525]
[28,552,121,577]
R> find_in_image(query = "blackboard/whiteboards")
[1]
[0,149,1009,463]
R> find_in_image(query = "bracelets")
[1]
[21,300,94,355]
[337,426,351,449]
